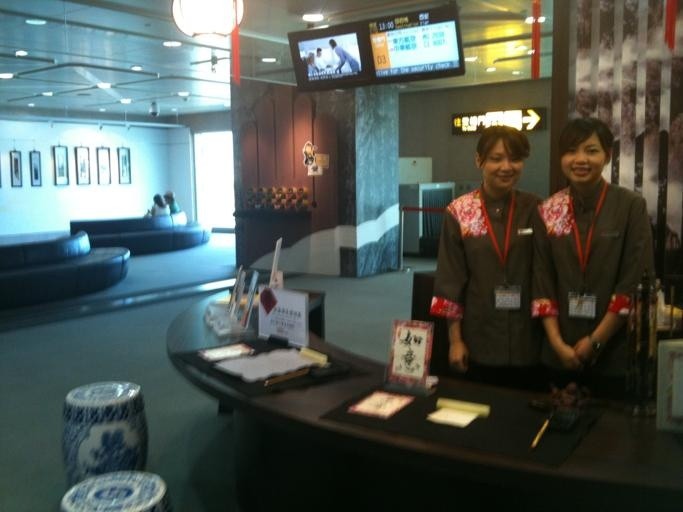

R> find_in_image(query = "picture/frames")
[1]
[52,144,69,187]
[97,146,112,186]
[29,150,42,186]
[117,145,132,186]
[75,145,90,185]
[10,150,23,188]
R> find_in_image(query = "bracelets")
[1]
[589,336,605,356]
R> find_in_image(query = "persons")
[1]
[314,48,333,70]
[164,191,181,215]
[329,39,359,74]
[144,194,171,216]
[429,125,545,387]
[306,52,325,77]
[531,116,656,404]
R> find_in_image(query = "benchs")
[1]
[0,229,129,313]
[71,214,210,252]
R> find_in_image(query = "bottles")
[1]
[625,281,658,417]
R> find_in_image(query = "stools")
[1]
[60,379,152,485]
[57,470,171,511]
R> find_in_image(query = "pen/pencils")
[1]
[530,419,550,449]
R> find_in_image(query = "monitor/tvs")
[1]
[287,22,373,91]
[363,3,466,92]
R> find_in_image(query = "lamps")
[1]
[171,0,244,63]
